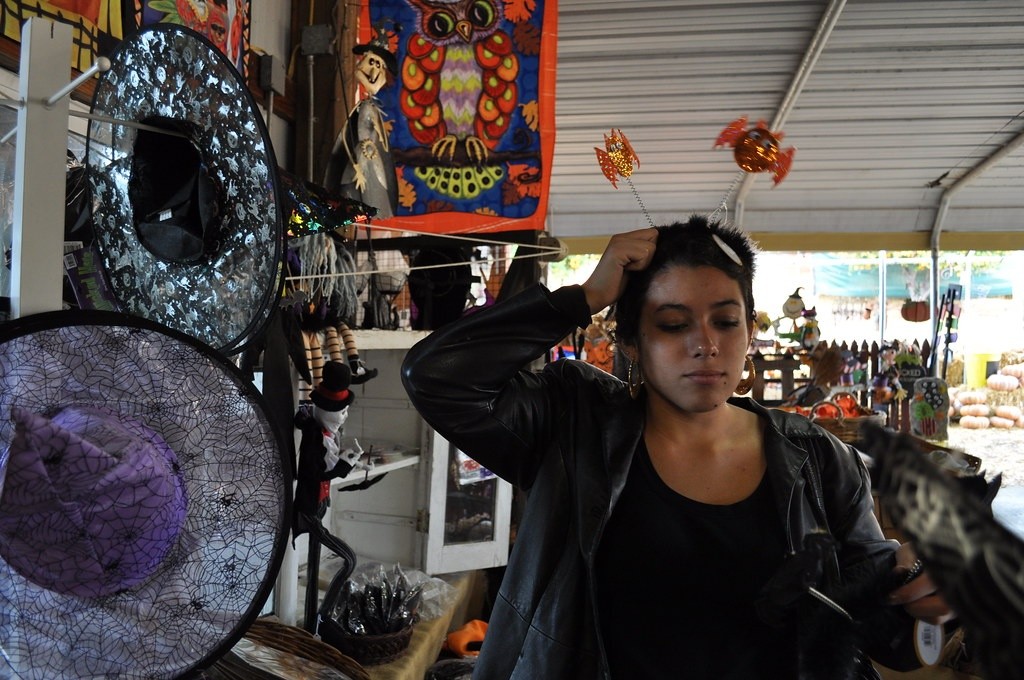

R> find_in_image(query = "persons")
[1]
[401,215,961,680]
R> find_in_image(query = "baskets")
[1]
[806,391,888,445]
[201,616,372,680]
[318,613,411,665]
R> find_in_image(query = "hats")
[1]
[86,30,380,358]
[0,309,292,678]
